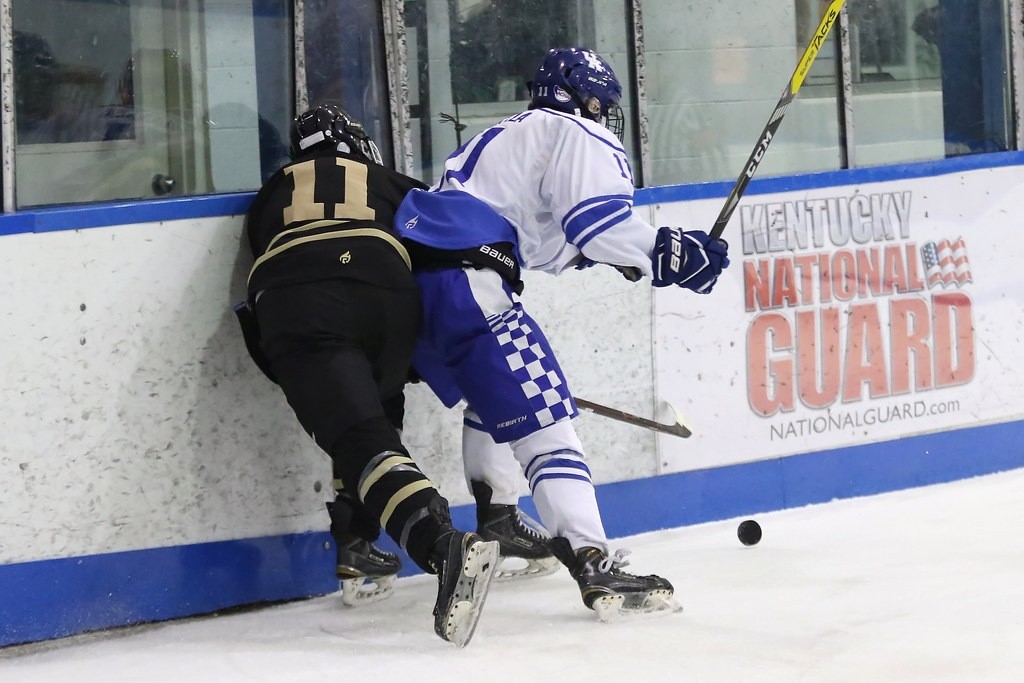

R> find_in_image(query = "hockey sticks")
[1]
[707,1,847,241]
[419,374,694,440]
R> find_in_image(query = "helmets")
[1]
[289,104,382,166]
[532,48,625,145]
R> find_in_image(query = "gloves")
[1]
[576,253,646,282]
[651,225,731,295]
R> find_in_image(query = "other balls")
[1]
[736,520,762,547]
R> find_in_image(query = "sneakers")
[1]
[427,495,500,647]
[326,501,402,607]
[547,533,684,623]
[467,478,562,581]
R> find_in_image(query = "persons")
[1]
[393,48,731,623]
[234,103,500,647]
